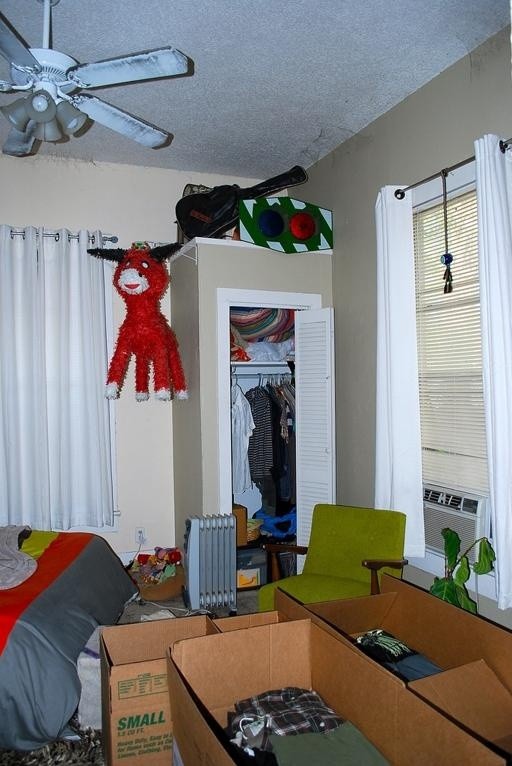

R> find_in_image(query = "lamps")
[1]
[0,90,89,143]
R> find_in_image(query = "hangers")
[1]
[233,373,295,403]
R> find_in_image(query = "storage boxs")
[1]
[238,197,334,255]
[101,573,512,766]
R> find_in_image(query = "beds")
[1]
[0,524,140,751]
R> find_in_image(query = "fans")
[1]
[0,0,189,157]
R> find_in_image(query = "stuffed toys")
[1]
[86,239,189,401]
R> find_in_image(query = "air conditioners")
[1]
[423,483,494,576]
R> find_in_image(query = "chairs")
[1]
[259,504,408,612]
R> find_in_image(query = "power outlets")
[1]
[135,527,145,541]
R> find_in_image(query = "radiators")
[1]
[183,514,239,618]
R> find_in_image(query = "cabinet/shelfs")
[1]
[216,286,336,592]
